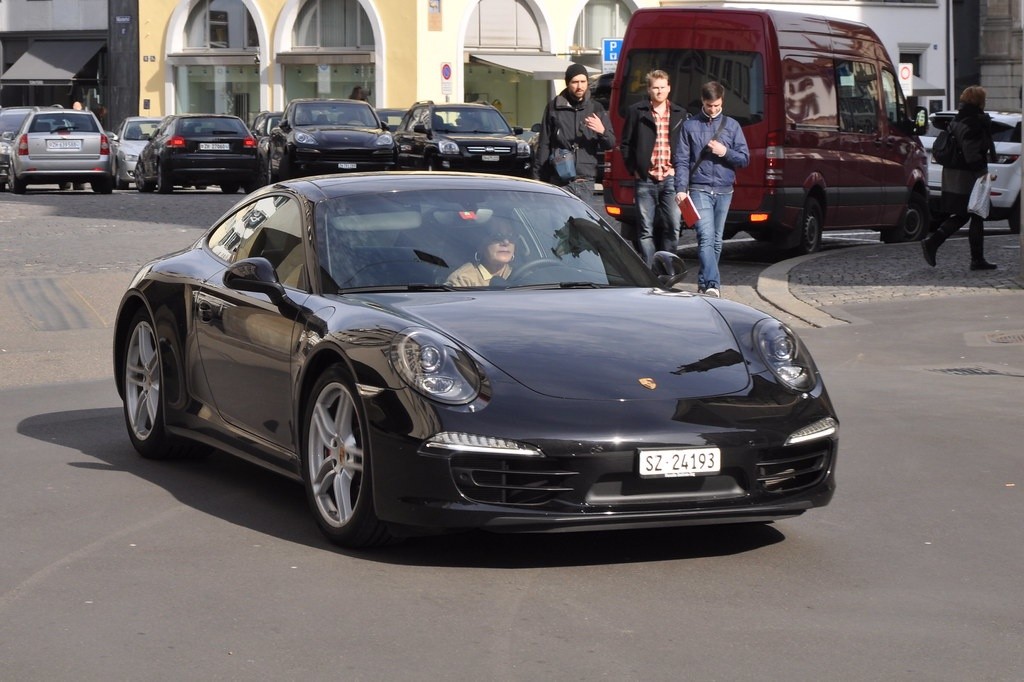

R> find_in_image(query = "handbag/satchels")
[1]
[970,173,994,213]
[551,148,575,181]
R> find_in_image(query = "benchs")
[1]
[310,114,336,124]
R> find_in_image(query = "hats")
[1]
[563,63,591,85]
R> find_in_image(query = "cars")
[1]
[0,104,538,186]
[2,105,112,195]
[134,114,262,193]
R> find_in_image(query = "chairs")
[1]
[432,114,444,129]
[298,110,311,123]
[73,121,90,132]
[32,121,51,133]
[335,113,352,124]
[128,126,143,140]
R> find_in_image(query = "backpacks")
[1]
[932,124,958,164]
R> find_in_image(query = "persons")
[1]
[533,65,615,208]
[445,215,516,287]
[348,86,365,101]
[678,196,701,226]
[96,107,106,126]
[675,82,750,298]
[621,69,687,270]
[923,85,997,270]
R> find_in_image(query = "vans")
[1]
[602,7,930,256]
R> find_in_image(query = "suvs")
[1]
[394,99,536,178]
[267,99,398,180]
[918,111,1022,234]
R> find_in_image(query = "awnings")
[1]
[896,68,945,97]
[0,40,106,86]
[469,52,603,81]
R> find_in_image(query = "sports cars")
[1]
[111,171,841,552]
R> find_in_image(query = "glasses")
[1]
[487,230,525,244]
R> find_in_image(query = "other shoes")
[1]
[968,256,999,269]
[705,287,724,299]
[921,236,938,267]
